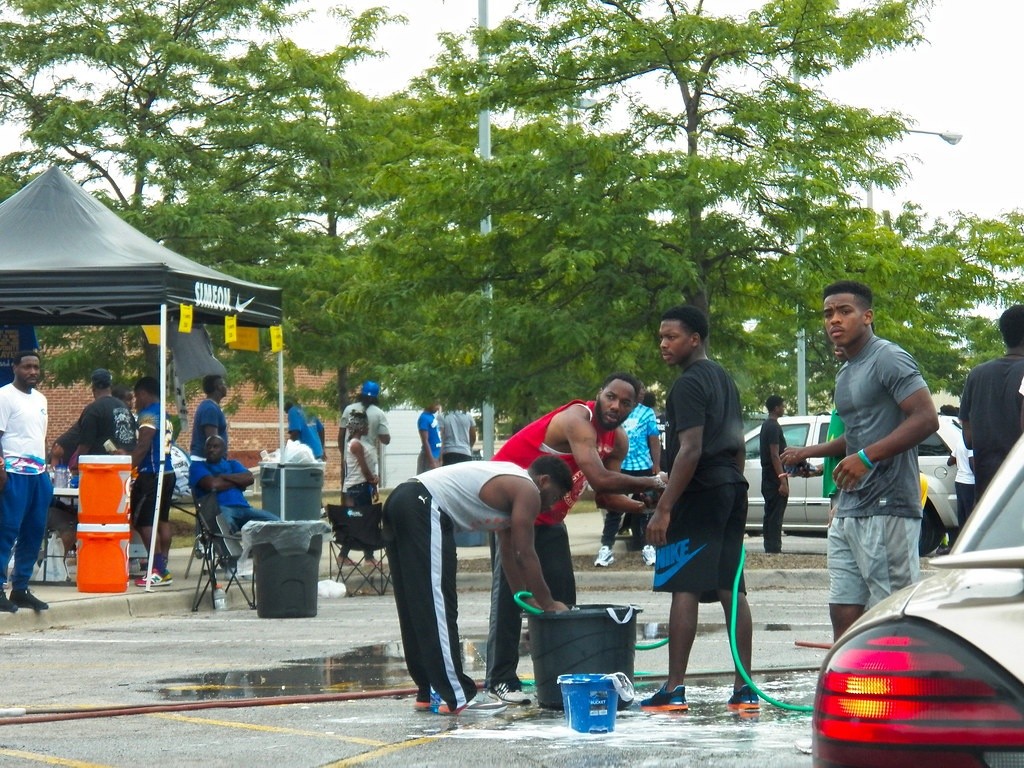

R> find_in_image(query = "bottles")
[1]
[54,465,68,488]
[260,450,270,462]
[213,584,228,612]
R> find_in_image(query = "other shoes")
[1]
[336,556,358,565]
[364,559,382,567]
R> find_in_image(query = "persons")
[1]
[0,280,1024,756]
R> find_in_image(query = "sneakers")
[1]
[414,701,430,711]
[488,682,532,704]
[594,545,615,567]
[9,590,49,610]
[642,544,656,565]
[437,692,508,715]
[640,681,688,711]
[727,684,761,710]
[135,569,173,587]
[0,591,19,611]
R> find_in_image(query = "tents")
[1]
[0,164,285,589]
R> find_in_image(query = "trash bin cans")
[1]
[243,522,331,618]
[258,462,325,521]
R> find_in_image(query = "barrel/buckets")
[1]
[520,604,643,711]
[77,455,133,593]
[558,674,619,733]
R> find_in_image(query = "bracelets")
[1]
[858,449,875,469]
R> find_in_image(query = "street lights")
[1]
[739,314,807,416]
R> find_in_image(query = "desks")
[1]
[44,488,79,582]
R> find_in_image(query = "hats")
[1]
[765,395,783,411]
[92,368,111,388]
[361,380,379,397]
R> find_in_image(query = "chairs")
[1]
[191,496,255,612]
[326,503,394,596]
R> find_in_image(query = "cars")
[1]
[743,412,968,557]
[811,430,1024,768]
